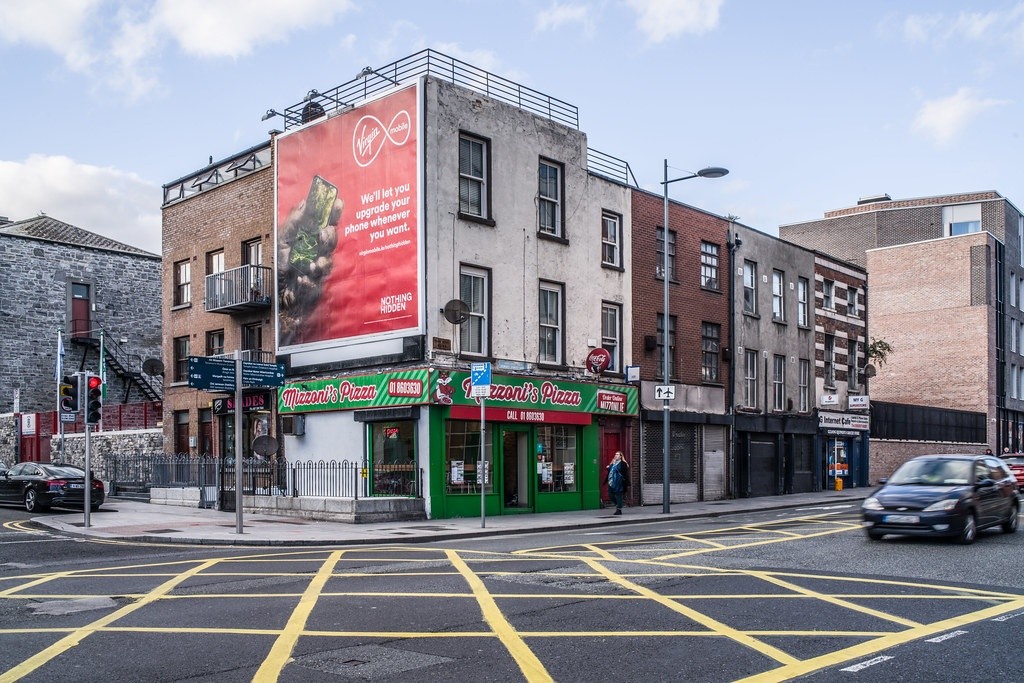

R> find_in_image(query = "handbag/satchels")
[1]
[622,477,631,494]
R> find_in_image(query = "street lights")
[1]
[659,158,729,514]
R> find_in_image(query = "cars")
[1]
[861,453,1021,544]
[998,453,1024,490]
[0,462,105,513]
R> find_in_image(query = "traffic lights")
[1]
[63,374,81,412]
[84,375,102,425]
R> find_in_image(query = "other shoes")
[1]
[614,509,622,515]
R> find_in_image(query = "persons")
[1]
[1002,447,1012,454]
[602,451,631,515]
[985,449,994,455]
[253,419,262,436]
[278,199,350,348]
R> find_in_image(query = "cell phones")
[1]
[288,174,338,275]
